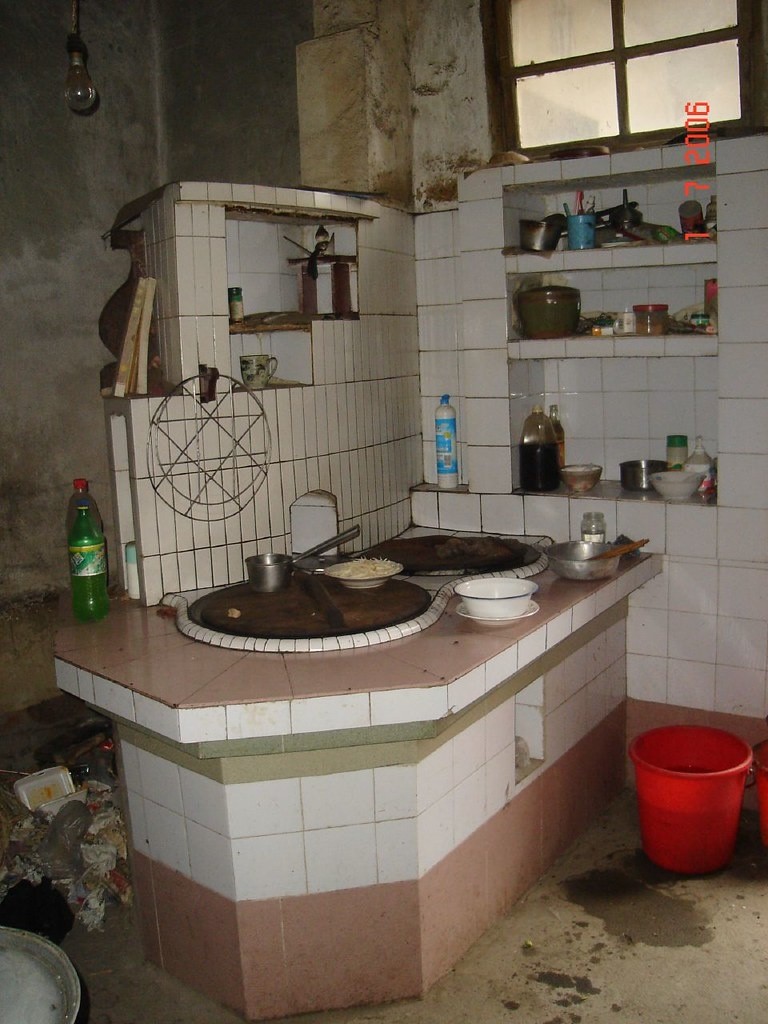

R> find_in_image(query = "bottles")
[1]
[228,287,245,325]
[665,434,714,492]
[434,395,565,493]
[65,480,140,622]
[705,194,717,242]
[633,305,668,336]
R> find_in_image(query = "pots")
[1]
[365,536,541,577]
[619,459,667,493]
[188,575,431,638]
[0,926,80,1024]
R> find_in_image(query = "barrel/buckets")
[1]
[628,725,768,875]
[518,219,561,252]
[516,284,582,338]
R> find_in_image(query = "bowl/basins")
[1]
[13,765,88,821]
[647,470,704,499]
[543,539,621,581]
[452,576,543,618]
[559,464,603,493]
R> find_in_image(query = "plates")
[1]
[456,599,540,625]
[323,559,405,590]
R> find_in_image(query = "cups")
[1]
[240,354,278,390]
[579,511,607,544]
[567,214,595,250]
